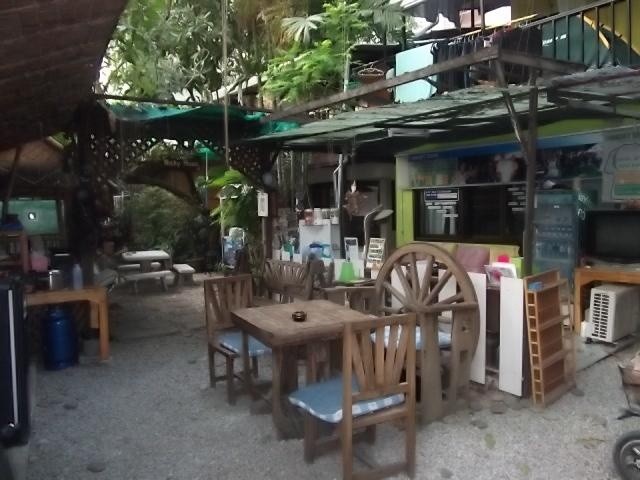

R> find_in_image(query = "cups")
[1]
[49,269,63,291]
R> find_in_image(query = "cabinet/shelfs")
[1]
[522,267,576,410]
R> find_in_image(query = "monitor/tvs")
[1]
[0,193,67,242]
[582,208,640,264]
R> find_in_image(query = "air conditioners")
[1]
[589,284,639,342]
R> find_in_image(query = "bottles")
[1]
[340,261,354,281]
[303,207,339,226]
[73,265,83,291]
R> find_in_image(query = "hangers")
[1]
[357,62,385,76]
[452,16,529,48]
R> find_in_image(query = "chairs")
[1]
[203,275,273,406]
[286,313,417,480]
[266,262,308,302]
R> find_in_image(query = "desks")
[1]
[227,296,380,441]
[23,285,109,363]
[121,250,171,271]
[572,263,639,335]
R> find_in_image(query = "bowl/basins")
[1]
[291,311,306,320]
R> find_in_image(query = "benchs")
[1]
[124,270,171,293]
[329,239,480,402]
[173,264,196,287]
[117,262,161,286]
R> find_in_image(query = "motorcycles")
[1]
[609,351,640,480]
